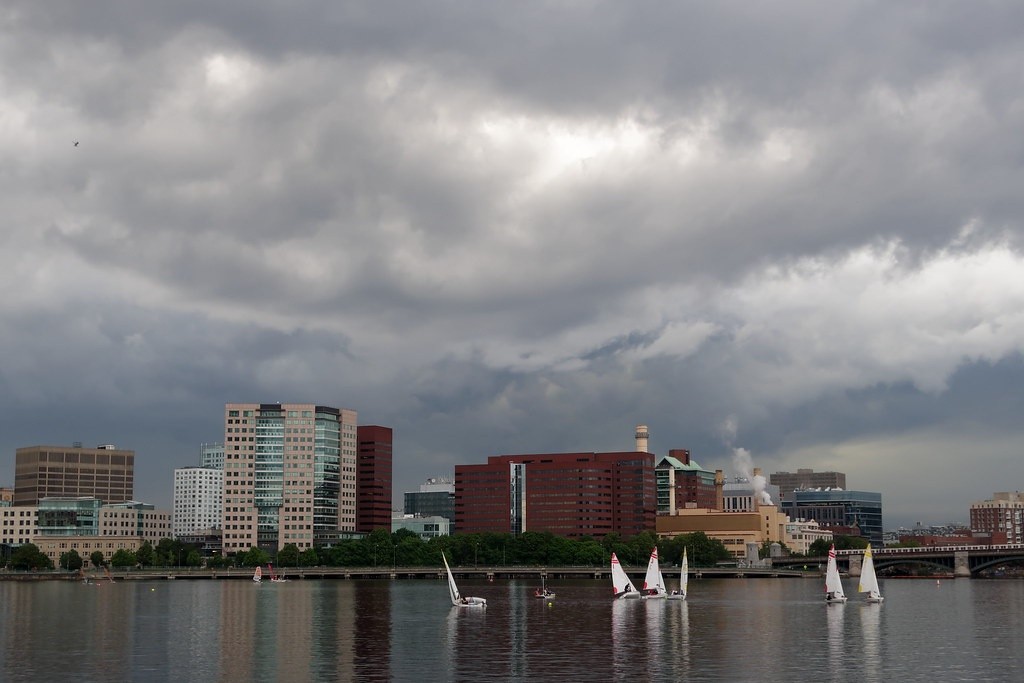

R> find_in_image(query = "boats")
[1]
[535,592,559,599]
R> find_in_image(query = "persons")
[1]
[826,592,832,600]
[534,588,539,595]
[546,586,552,595]
[136,560,142,575]
[462,597,468,604]
[625,583,629,592]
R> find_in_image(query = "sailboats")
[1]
[611,552,641,599]
[253,566,263,584]
[269,564,287,583]
[641,546,669,599]
[824,542,848,604]
[441,549,488,607]
[856,543,885,604]
[665,546,688,600]
